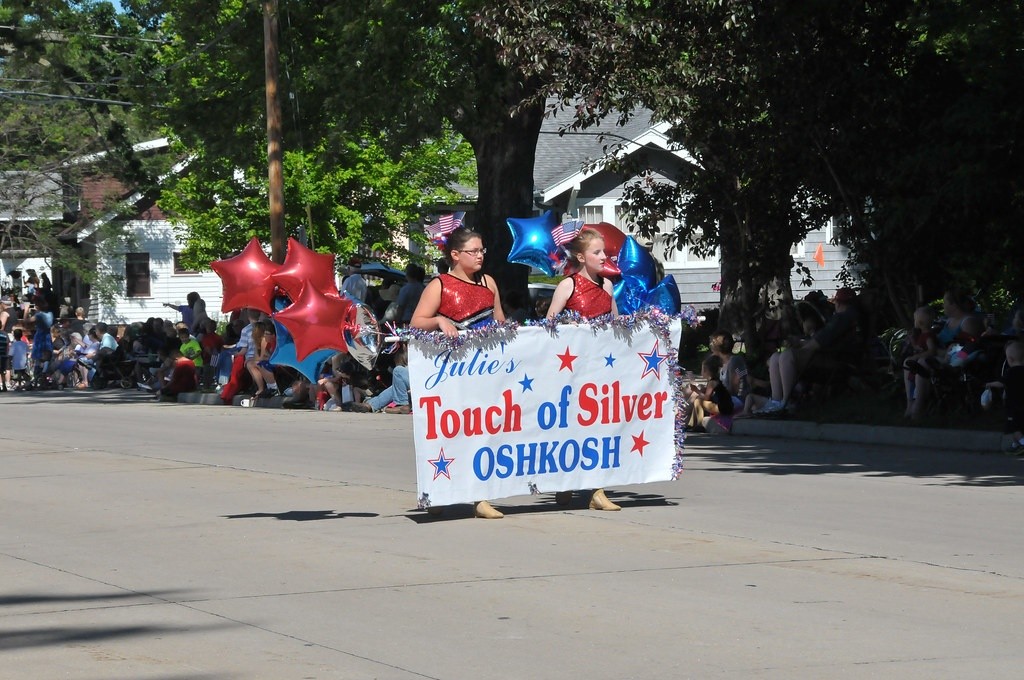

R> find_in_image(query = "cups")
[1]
[241,399,249,407]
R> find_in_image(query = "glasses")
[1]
[458,248,487,255]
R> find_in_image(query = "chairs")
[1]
[785,315,983,428]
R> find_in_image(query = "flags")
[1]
[440,211,468,234]
[550,220,585,246]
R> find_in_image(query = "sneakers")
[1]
[753,400,783,418]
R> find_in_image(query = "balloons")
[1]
[211,237,380,372]
[564,221,681,318]
[507,210,561,278]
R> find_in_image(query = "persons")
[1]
[0,269,281,401]
[409,227,506,519]
[318,344,415,414]
[337,257,451,334]
[897,290,1024,456]
[676,287,870,433]
[545,228,622,511]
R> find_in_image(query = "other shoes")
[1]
[687,426,705,433]
[1005,437,1024,455]
[905,360,930,379]
[555,492,572,505]
[732,413,752,419]
[473,502,504,519]
[235,386,413,414]
[589,488,622,511]
[428,507,445,515]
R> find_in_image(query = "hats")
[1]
[348,258,362,268]
[379,284,401,301]
[830,288,856,302]
